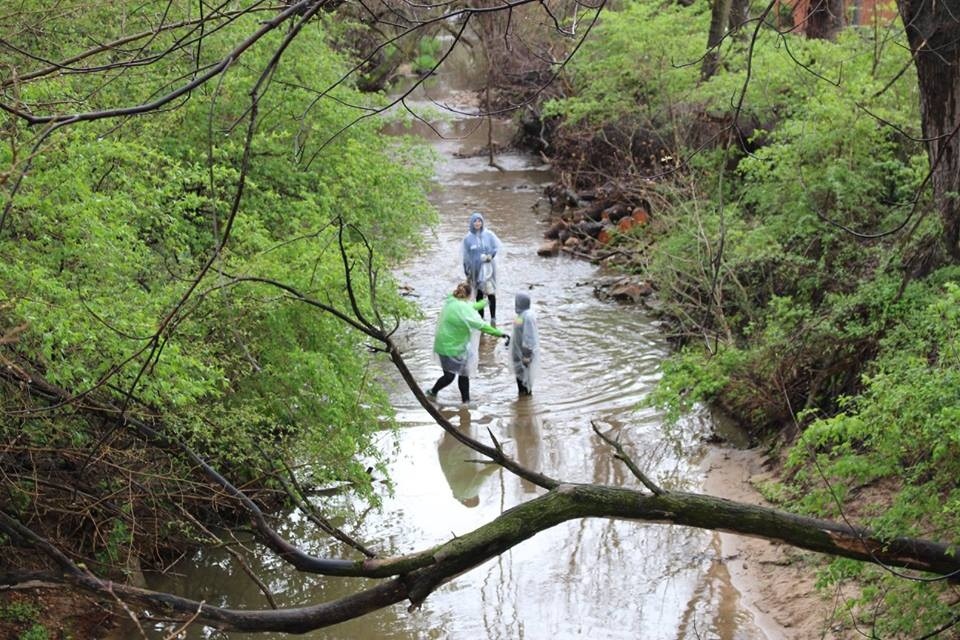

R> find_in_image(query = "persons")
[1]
[463,212,502,330]
[426,282,511,406]
[511,292,540,398]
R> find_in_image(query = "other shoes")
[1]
[425,390,437,403]
